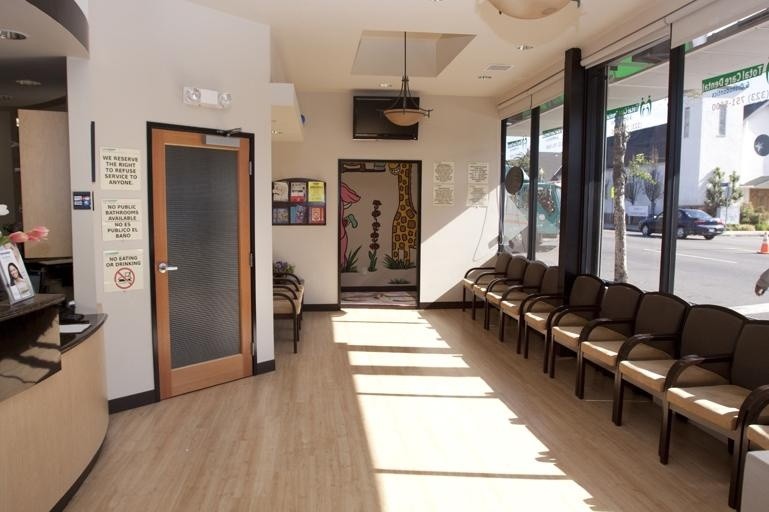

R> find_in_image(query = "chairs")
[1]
[273,272,305,353]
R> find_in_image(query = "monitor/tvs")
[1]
[353,96,420,139]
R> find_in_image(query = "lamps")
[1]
[488,0,570,21]
[376,31,433,126]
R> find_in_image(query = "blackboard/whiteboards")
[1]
[19,108,72,258]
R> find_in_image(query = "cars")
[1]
[638,209,726,241]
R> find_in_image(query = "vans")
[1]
[508,183,562,252]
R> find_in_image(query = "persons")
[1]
[8,262,30,298]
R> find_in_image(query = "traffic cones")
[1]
[757,230,768,255]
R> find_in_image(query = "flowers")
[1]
[0,204,50,246]
